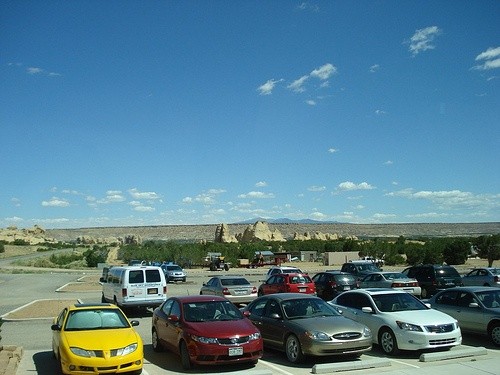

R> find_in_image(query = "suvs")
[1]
[401,264,464,299]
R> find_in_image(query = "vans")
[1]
[99,265,167,314]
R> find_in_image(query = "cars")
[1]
[416,285,500,347]
[461,267,500,287]
[311,270,362,301]
[341,256,385,280]
[265,266,305,283]
[359,272,422,299]
[257,272,316,298]
[50,302,144,375]
[151,294,264,371]
[238,292,374,364]
[127,259,187,282]
[326,287,463,356]
[199,275,259,306]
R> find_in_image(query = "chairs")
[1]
[185,307,202,322]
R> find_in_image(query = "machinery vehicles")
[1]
[206,251,232,271]
